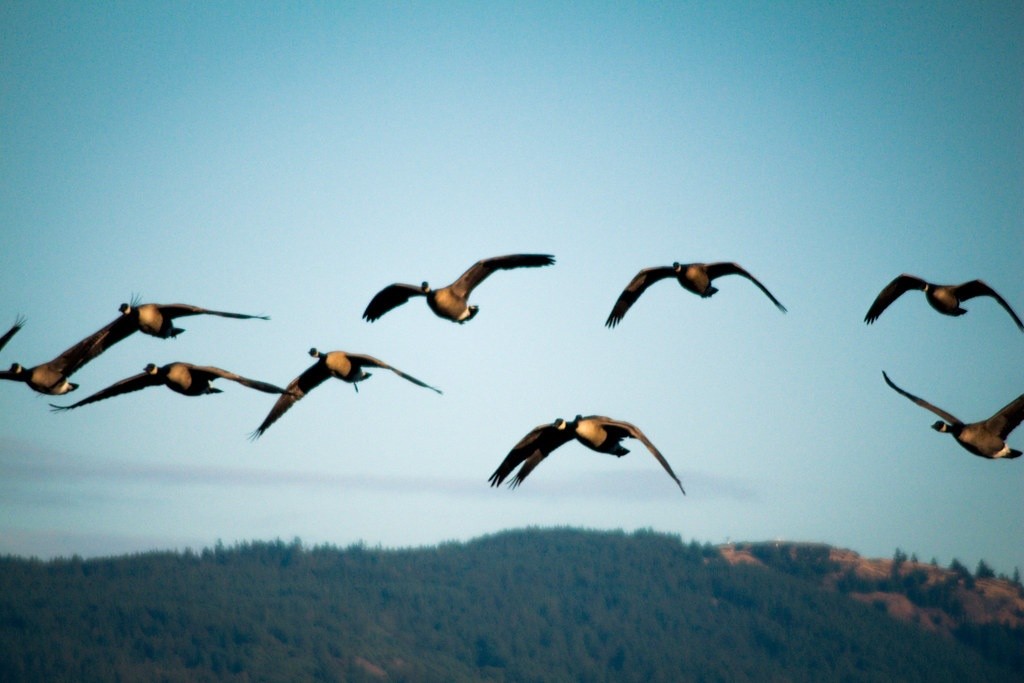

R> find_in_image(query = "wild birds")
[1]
[0,251,1023,500]
